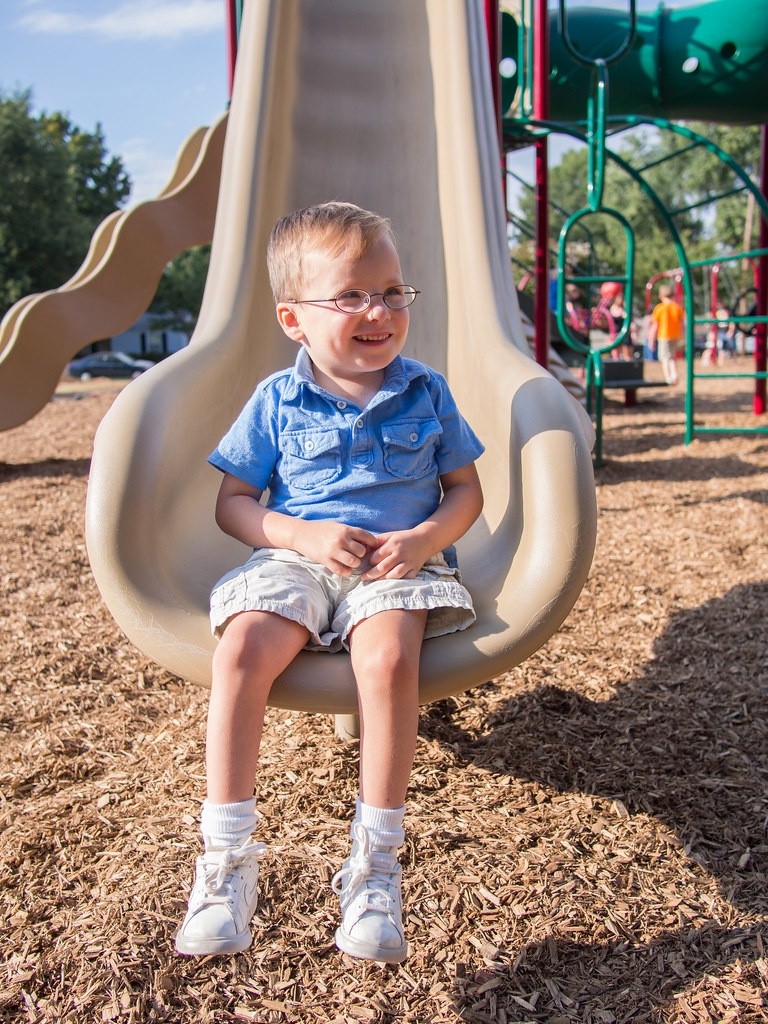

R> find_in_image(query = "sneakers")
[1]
[175,836,266,955]
[331,823,407,963]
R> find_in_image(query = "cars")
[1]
[69,350,155,384]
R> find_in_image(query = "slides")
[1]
[83,0,600,716]
[0,113,231,437]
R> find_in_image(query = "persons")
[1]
[177,202,484,964]
[566,282,757,387]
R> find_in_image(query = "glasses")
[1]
[287,284,422,314]
